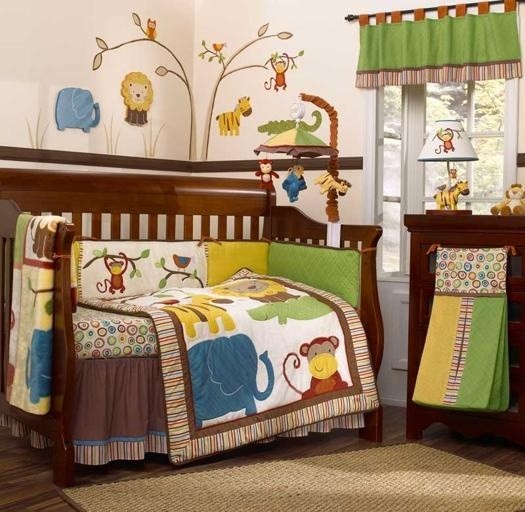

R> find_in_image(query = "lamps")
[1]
[420,118,481,215]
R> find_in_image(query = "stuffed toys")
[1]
[433,179,469,210]
[314,171,351,197]
[491,184,525,216]
[255,159,278,190]
[282,164,307,202]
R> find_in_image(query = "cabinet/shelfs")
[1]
[403,200,525,447]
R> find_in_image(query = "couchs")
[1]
[0,168,388,486]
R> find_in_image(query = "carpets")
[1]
[56,443,525,512]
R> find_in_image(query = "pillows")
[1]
[268,237,362,308]
[199,234,270,286]
[71,237,207,302]
